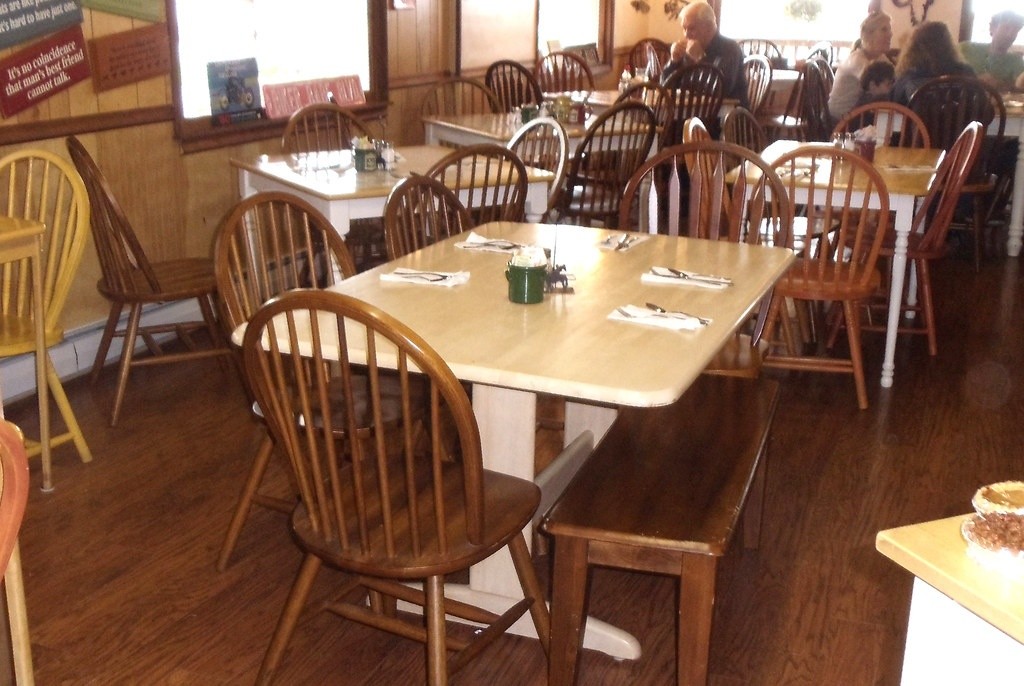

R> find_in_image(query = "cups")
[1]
[522,103,539,123]
[635,67,648,96]
[854,138,876,164]
[352,145,386,172]
[557,95,571,123]
[569,101,592,126]
[505,260,547,304]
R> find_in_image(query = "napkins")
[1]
[600,233,650,252]
[642,266,729,290]
[607,304,711,332]
[880,165,938,172]
[380,267,470,288]
[453,231,526,254]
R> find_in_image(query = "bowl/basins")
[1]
[962,479,1024,584]
[1003,101,1024,113]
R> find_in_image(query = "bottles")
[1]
[506,106,521,124]
[830,132,856,160]
[618,65,631,94]
[373,138,396,170]
[540,101,554,118]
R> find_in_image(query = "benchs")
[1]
[536,371,783,686]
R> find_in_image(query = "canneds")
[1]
[555,95,572,124]
[568,102,585,125]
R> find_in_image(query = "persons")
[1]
[956,12,1024,88]
[823,11,894,136]
[893,21,994,239]
[847,61,896,131]
[660,0,749,145]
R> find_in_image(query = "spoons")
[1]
[645,301,708,326]
[667,267,733,286]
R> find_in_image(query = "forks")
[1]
[649,267,720,286]
[616,306,686,321]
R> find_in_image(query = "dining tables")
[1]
[745,67,803,120]
[876,90,1023,258]
[875,512,1024,686]
[0,214,56,494]
[228,144,556,306]
[420,113,666,223]
[229,219,797,664]
[723,139,947,387]
[541,90,740,142]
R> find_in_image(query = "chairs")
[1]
[0,148,94,465]
[209,190,459,571]
[826,119,985,358]
[279,103,390,289]
[617,140,791,380]
[382,176,477,464]
[240,289,552,686]
[65,132,236,429]
[425,38,1023,272]
[748,146,892,410]
